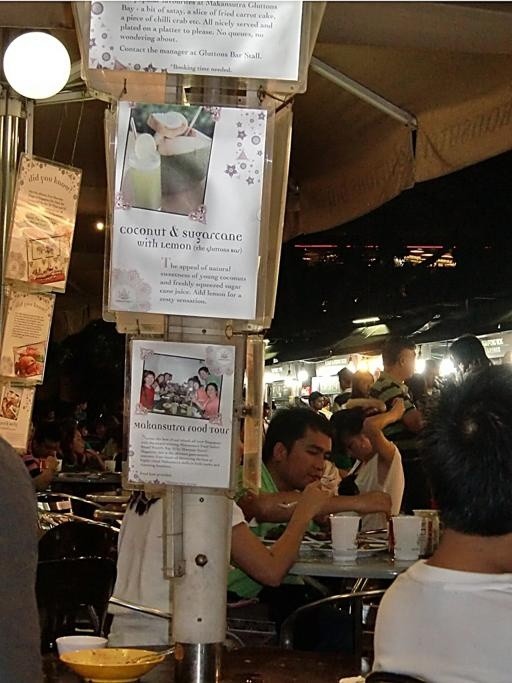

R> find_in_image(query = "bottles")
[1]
[412,508,441,558]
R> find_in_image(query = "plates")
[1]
[255,532,329,553]
[311,541,387,560]
[59,648,165,682]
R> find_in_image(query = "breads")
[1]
[20,346,40,357]
[17,363,43,376]
[18,356,35,368]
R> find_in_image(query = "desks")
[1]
[35,464,420,682]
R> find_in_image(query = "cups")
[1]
[389,515,424,560]
[330,515,359,562]
[55,635,108,656]
[331,560,361,571]
[392,559,418,571]
[55,459,62,472]
[105,460,115,472]
[128,153,163,212]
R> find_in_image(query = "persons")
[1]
[229,333,511,627]
[0,436,46,682]
[371,363,512,681]
[36,252,69,283]
[140,365,218,418]
[27,397,120,502]
[108,477,333,680]
[6,386,22,405]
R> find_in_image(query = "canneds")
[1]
[415,510,439,556]
